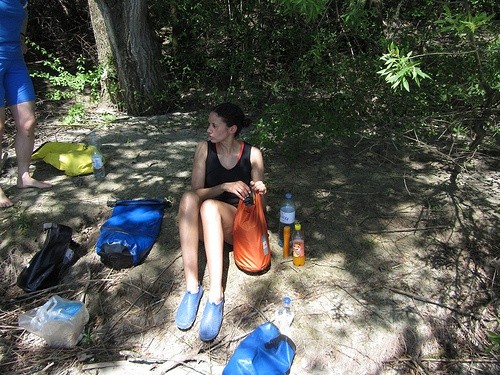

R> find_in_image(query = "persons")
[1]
[176,103,268,341]
[0,0,52,208]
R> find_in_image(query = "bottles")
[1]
[63,248,74,267]
[293,223,305,266]
[278,193,295,247]
[277,297,292,335]
[284,226,292,259]
[89,131,105,180]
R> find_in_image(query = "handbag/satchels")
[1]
[31,140,106,177]
[222,321,297,375]
[15,222,80,292]
[97,199,168,269]
[233,189,271,273]
[18,295,90,346]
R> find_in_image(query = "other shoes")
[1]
[175,285,203,329]
[198,297,224,341]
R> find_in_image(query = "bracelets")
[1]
[20,32,26,37]
